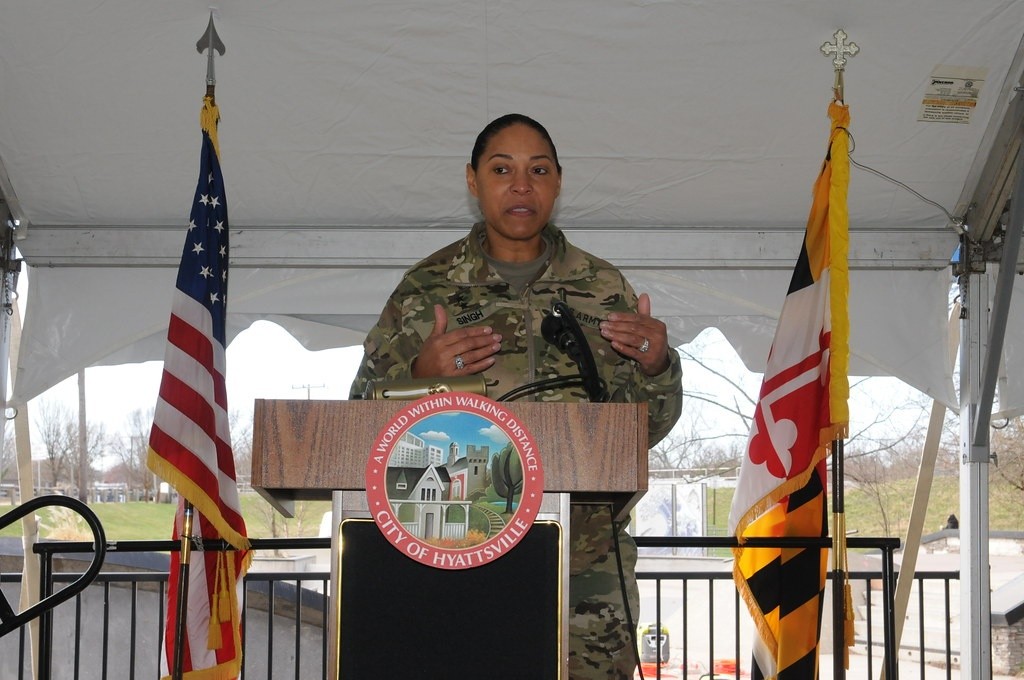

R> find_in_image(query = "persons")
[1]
[349,114,684,680]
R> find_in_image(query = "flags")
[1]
[146,90,252,680]
[728,103,849,678]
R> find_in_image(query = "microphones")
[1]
[541,314,585,362]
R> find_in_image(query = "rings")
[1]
[455,356,464,370]
[638,339,649,352]
